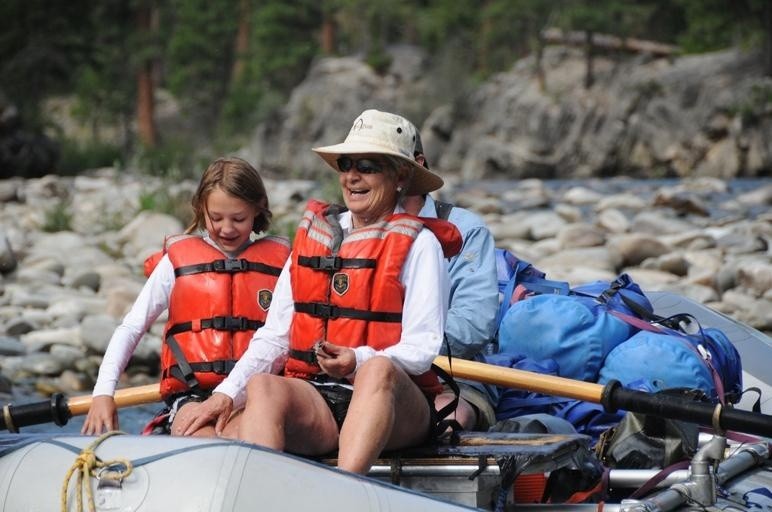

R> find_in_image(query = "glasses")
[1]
[336,157,383,174]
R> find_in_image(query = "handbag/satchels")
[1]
[607,391,704,469]
[498,281,653,382]
[597,323,742,404]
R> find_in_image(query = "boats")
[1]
[3,278,771,511]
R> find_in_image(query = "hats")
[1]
[311,109,444,196]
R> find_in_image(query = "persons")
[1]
[81,157,292,441]
[400,130,501,431]
[174,109,462,478]
